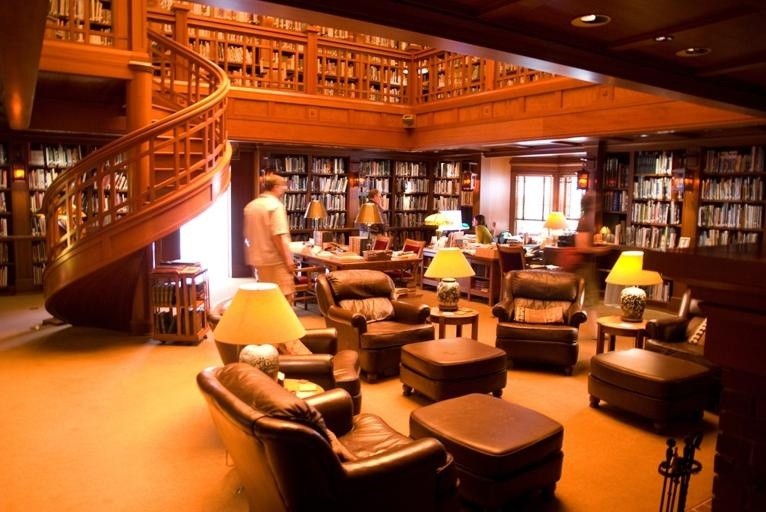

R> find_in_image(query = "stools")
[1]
[397,337,507,403]
[408,393,565,511]
[587,347,708,435]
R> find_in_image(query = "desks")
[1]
[281,378,324,401]
[426,306,479,340]
[595,316,648,356]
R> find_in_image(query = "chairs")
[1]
[195,362,461,512]
[204,297,362,414]
[313,268,435,385]
[643,288,706,364]
[289,263,324,312]
[496,244,547,271]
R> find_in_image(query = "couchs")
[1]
[492,269,589,375]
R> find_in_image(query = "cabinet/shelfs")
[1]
[149,266,212,345]
[420,244,501,307]
[594,127,765,260]
[232,145,482,277]
[48,2,563,104]
[0,133,128,294]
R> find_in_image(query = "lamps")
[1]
[423,247,476,312]
[543,212,569,246]
[575,158,589,191]
[209,283,307,382]
[603,251,663,323]
